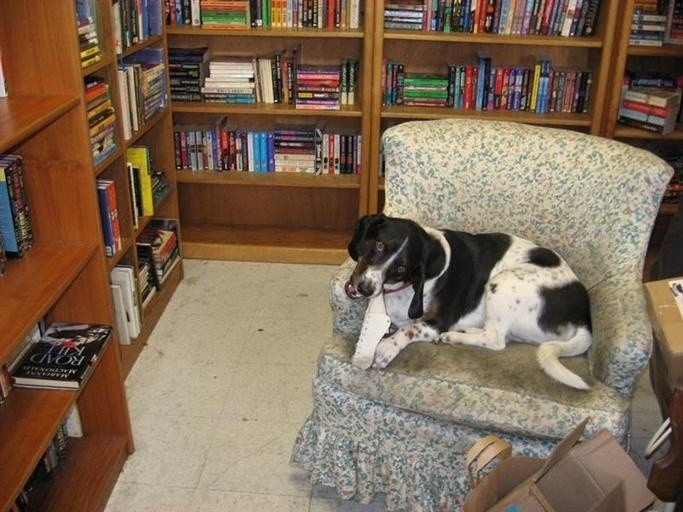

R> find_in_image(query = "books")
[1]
[0,318,112,405]
[385,0,599,37]
[74,0,184,346]
[162,0,361,30]
[660,151,683,201]
[0,154,34,277]
[9,403,83,512]
[382,52,593,114]
[628,0,683,47]
[617,70,683,135]
[173,115,361,175]
[168,42,356,110]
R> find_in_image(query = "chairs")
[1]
[312,117,674,511]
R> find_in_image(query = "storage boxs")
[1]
[645,277,683,456]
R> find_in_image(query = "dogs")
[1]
[342,210,595,393]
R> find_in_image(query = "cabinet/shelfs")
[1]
[371,0,611,278]
[164,3,375,268]
[615,8,682,275]
[0,0,185,512]
